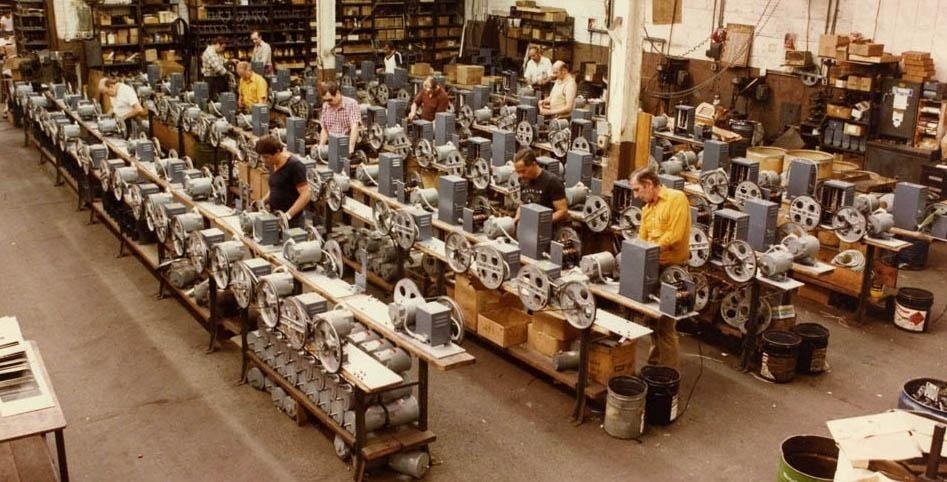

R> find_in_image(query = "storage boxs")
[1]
[238,160,271,203]
[818,35,902,64]
[785,50,812,68]
[797,282,831,306]
[151,117,198,162]
[454,272,635,387]
[411,62,485,84]
[507,0,568,42]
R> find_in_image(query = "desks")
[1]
[0,341,69,482]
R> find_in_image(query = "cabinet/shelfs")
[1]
[81,0,190,89]
[503,16,575,72]
[193,0,464,73]
[816,62,947,198]
[0,0,62,103]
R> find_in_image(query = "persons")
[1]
[248,30,274,78]
[97,77,145,141]
[0,10,14,39]
[522,45,554,97]
[627,165,693,369]
[407,75,449,124]
[511,146,567,226]
[234,60,270,114]
[379,40,403,75]
[318,81,363,227]
[536,60,577,128]
[253,134,311,296]
[198,36,236,104]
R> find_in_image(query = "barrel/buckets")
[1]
[894,287,934,333]
[603,376,648,440]
[641,365,680,425]
[775,434,839,482]
[760,329,802,383]
[793,322,830,373]
[897,378,947,423]
